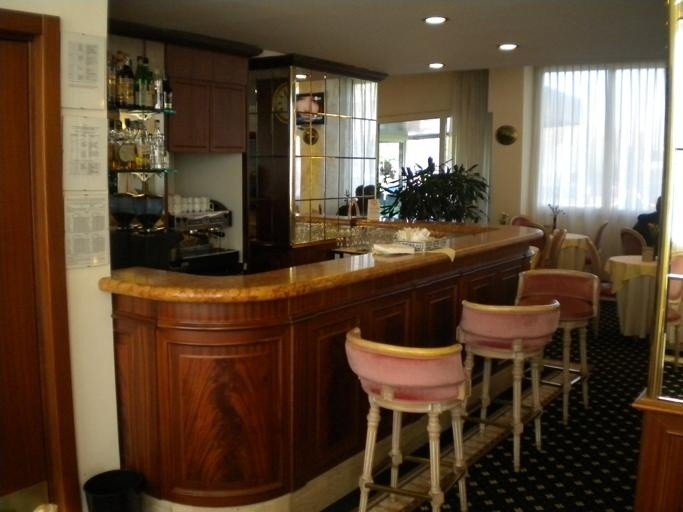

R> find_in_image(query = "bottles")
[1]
[144,57,154,107]
[108,120,165,170]
[118,56,134,107]
[154,66,163,109]
[135,56,143,106]
[163,84,173,109]
[107,53,117,107]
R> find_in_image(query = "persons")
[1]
[632,196,662,249]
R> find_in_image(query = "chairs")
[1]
[588,221,608,265]
[456,297,560,471]
[543,228,568,268]
[586,238,604,274]
[343,325,465,512]
[620,226,645,256]
[528,224,550,268]
[510,214,532,226]
[516,270,599,411]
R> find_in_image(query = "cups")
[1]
[168,195,214,217]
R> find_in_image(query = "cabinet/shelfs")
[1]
[246,54,387,271]
[167,40,247,154]
[108,101,177,270]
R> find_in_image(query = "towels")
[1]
[374,241,414,255]
